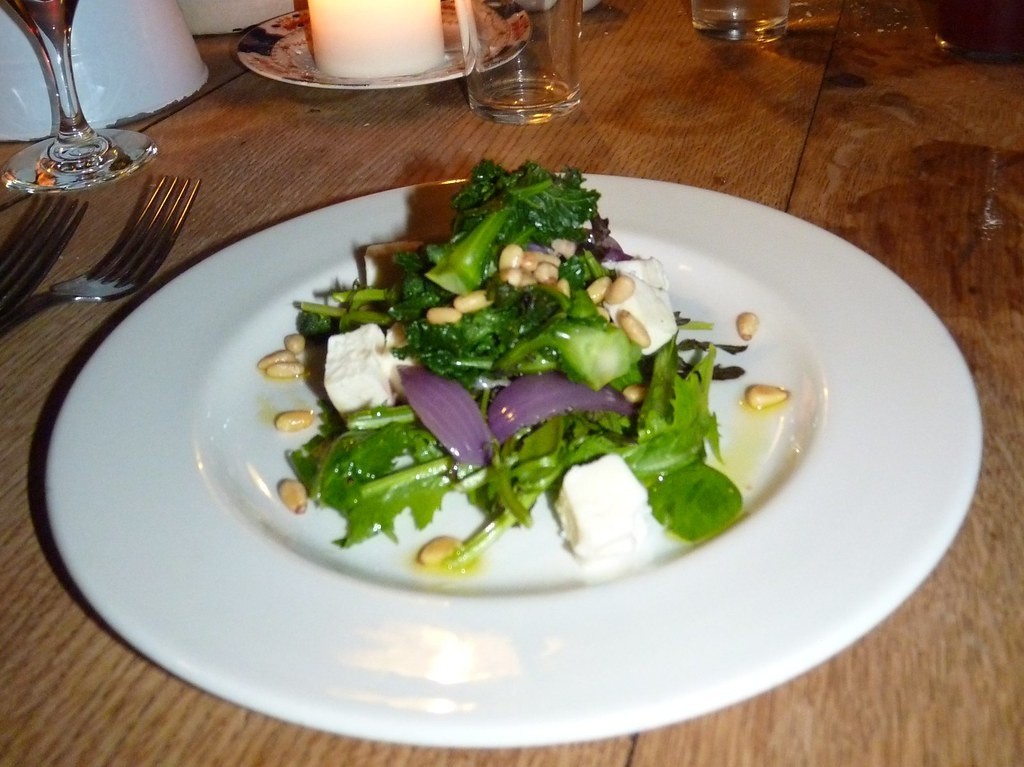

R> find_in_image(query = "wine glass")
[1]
[0,0,159,193]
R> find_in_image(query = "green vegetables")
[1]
[294,157,744,569]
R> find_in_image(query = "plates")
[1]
[46,173,983,749]
[237,1,531,90]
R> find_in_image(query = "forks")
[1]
[0,195,89,320]
[0,176,201,339]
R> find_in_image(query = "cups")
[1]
[454,0,583,124]
[693,1,790,43]
